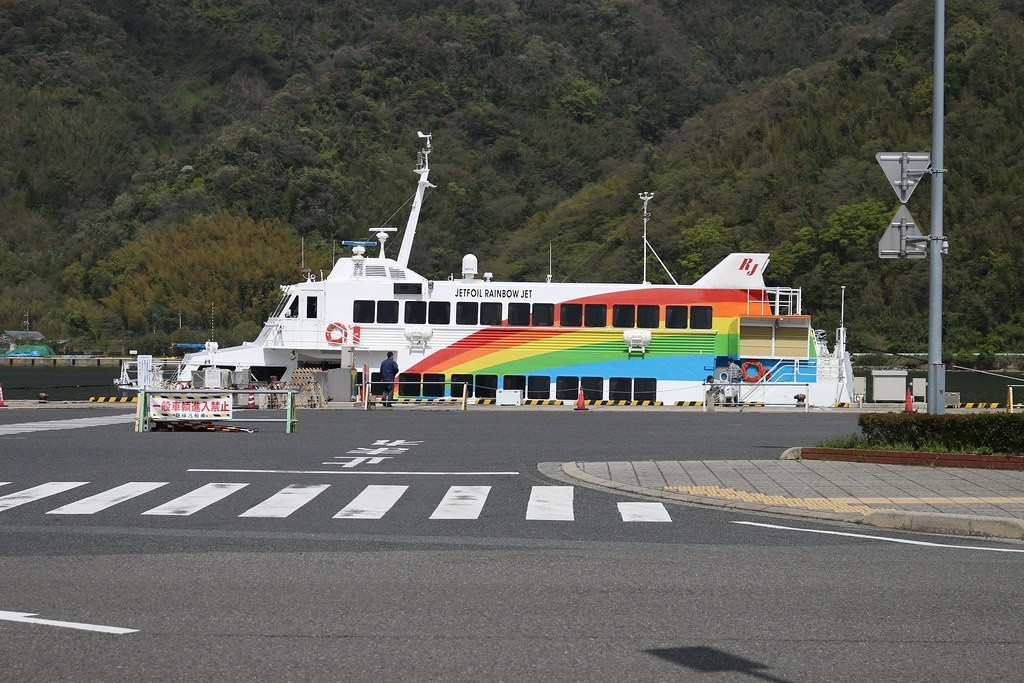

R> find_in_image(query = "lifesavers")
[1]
[741,360,762,383]
[325,323,347,343]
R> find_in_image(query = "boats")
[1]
[111,127,961,411]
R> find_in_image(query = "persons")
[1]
[380,352,399,407]
[725,358,744,407]
[700,375,725,407]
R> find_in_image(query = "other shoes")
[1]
[731,404,738,407]
[723,404,730,407]
[387,404,392,407]
[382,397,386,406]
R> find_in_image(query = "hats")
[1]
[707,376,712,382]
